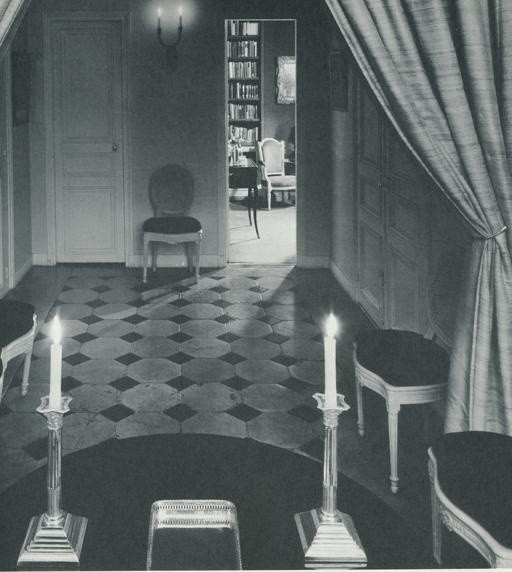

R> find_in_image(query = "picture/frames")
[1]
[275,56,297,104]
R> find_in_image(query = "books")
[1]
[227,19,259,145]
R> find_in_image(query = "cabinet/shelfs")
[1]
[225,21,261,161]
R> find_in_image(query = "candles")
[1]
[323,336,337,406]
[50,345,63,404]
[156,19,162,29]
[179,16,183,27]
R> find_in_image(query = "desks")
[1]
[229,157,261,241]
[4,433,439,570]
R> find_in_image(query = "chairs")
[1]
[427,430,512,570]
[353,243,473,494]
[140,162,204,285]
[257,134,297,210]
